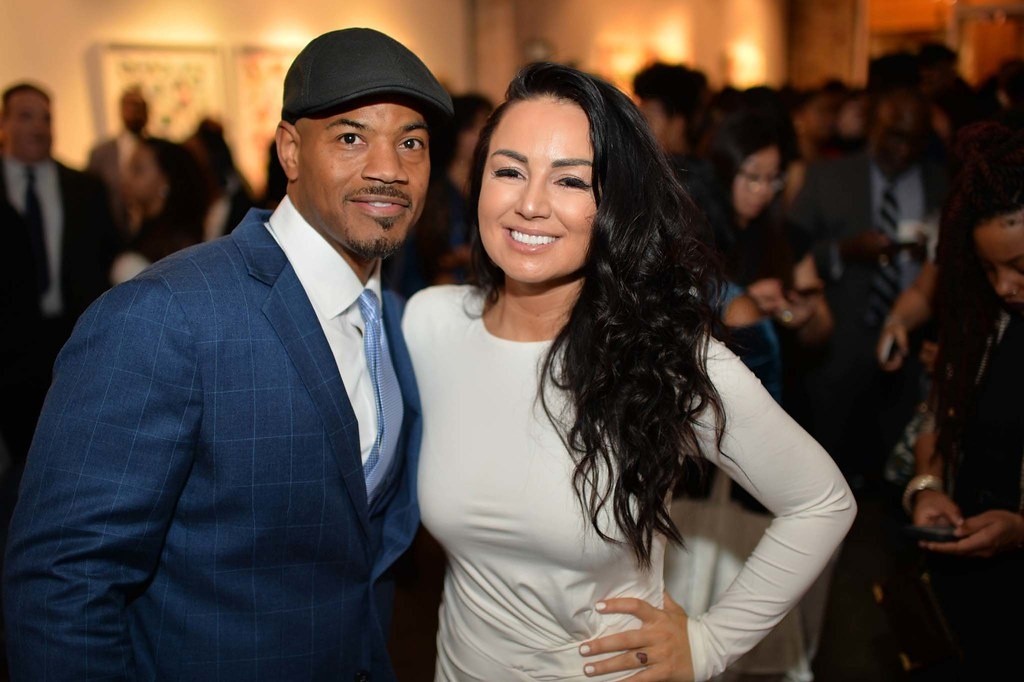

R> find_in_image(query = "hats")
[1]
[282,28,454,137]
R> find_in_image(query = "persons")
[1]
[1,27,458,682]
[403,80,504,307]
[0,77,291,556]
[400,27,860,681]
[625,40,1024,680]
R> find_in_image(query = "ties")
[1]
[355,289,405,501]
[22,165,53,313]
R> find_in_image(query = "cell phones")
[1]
[881,335,899,364]
[795,287,821,299]
[904,523,971,541]
